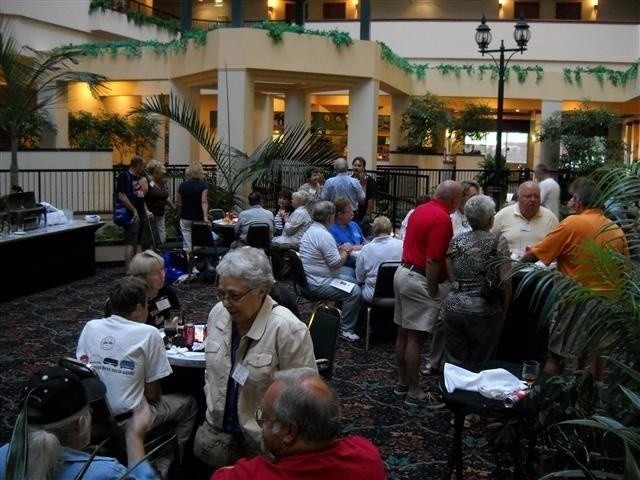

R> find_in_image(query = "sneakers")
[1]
[404,391,446,410]
[394,382,429,396]
[338,329,361,341]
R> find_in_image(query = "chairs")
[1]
[59,357,180,470]
[147,209,402,352]
[308,303,343,383]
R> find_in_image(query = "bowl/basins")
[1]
[86,215,101,222]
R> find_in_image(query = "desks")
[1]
[0,220,106,305]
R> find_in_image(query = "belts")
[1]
[400,261,426,279]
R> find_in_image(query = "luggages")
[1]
[157,246,189,283]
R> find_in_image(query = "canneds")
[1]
[504,394,519,408]
[203,324,208,341]
[225,211,233,221]
[183,323,195,345]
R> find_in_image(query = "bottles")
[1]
[504,389,528,408]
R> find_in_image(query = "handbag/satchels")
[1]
[192,419,239,468]
[113,171,133,228]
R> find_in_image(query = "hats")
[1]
[18,367,108,427]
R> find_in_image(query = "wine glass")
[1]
[162,309,183,342]
[522,361,539,389]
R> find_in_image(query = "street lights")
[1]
[474,13,532,213]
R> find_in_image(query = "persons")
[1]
[0,157,640,480]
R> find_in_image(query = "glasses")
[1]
[142,266,168,277]
[211,283,255,303]
[255,406,291,428]
